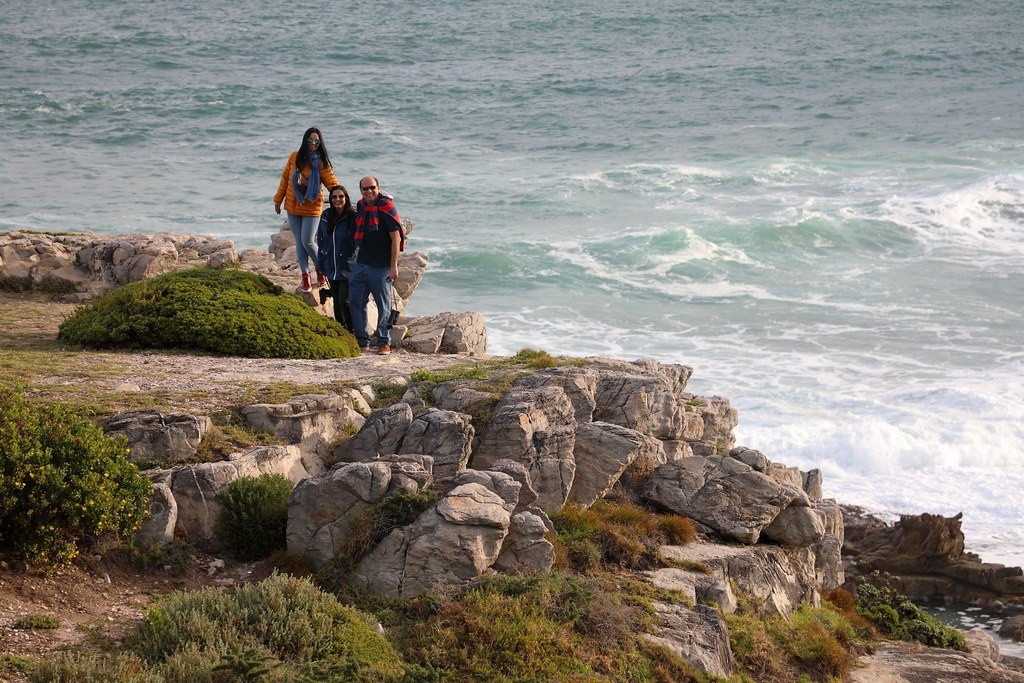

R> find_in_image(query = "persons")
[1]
[273,127,357,293]
[348,177,401,355]
[317,185,356,335]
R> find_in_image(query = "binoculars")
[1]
[386,310,400,330]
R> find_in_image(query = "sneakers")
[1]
[315,268,327,286]
[301,273,312,293]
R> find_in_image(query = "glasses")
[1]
[307,138,320,144]
[332,195,346,199]
[362,186,378,191]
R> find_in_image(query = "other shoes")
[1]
[378,344,390,355]
[361,346,370,350]
